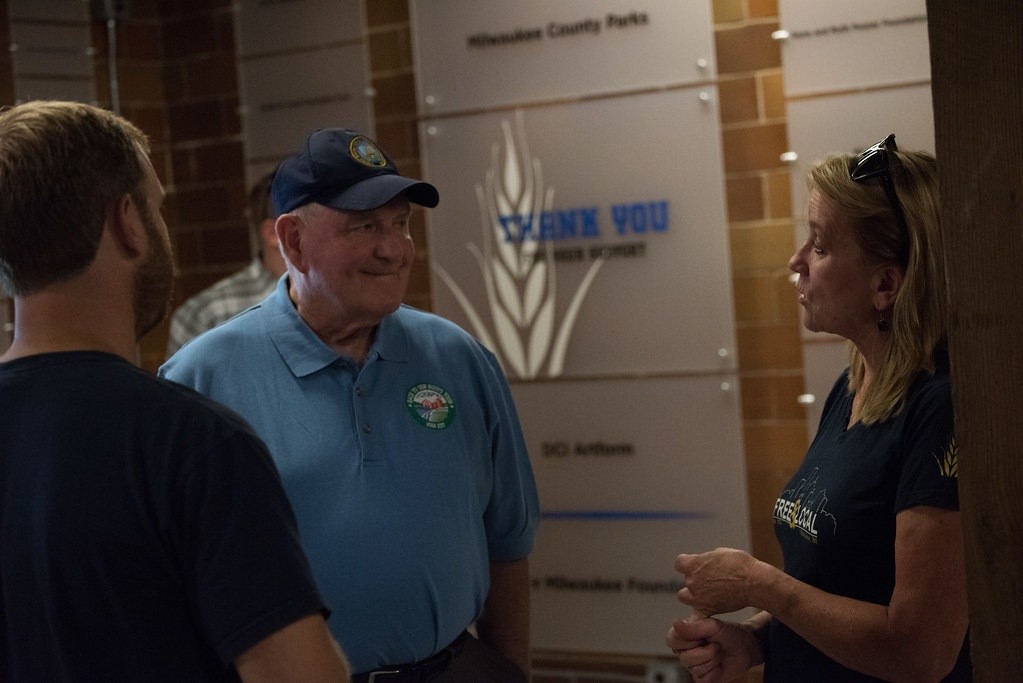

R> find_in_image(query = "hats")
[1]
[270,127,440,212]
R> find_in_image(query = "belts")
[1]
[352,629,468,682]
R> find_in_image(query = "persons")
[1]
[159,123,547,683]
[2,95,356,683]
[166,166,293,352]
[659,148,976,683]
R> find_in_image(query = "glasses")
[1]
[851,132,909,266]
